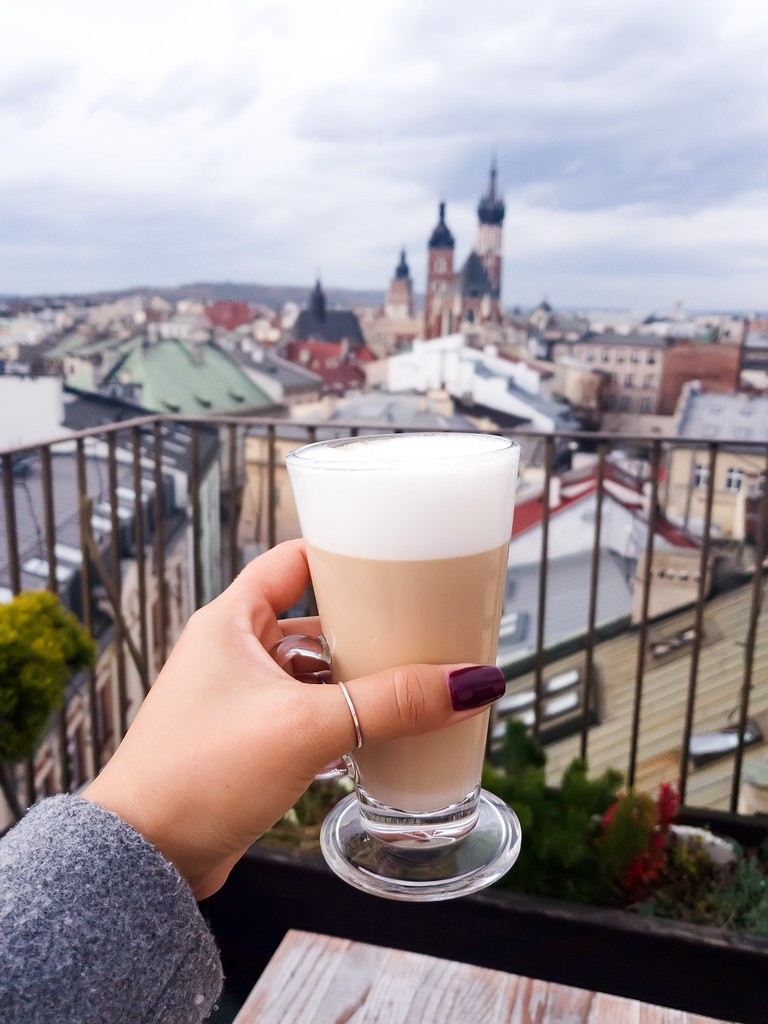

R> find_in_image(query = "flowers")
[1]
[0,585,99,765]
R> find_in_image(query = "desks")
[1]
[229,927,737,1024]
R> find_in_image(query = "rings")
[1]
[336,679,364,750]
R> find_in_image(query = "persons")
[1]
[1,532,507,1024]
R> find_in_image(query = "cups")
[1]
[268,433,524,905]
